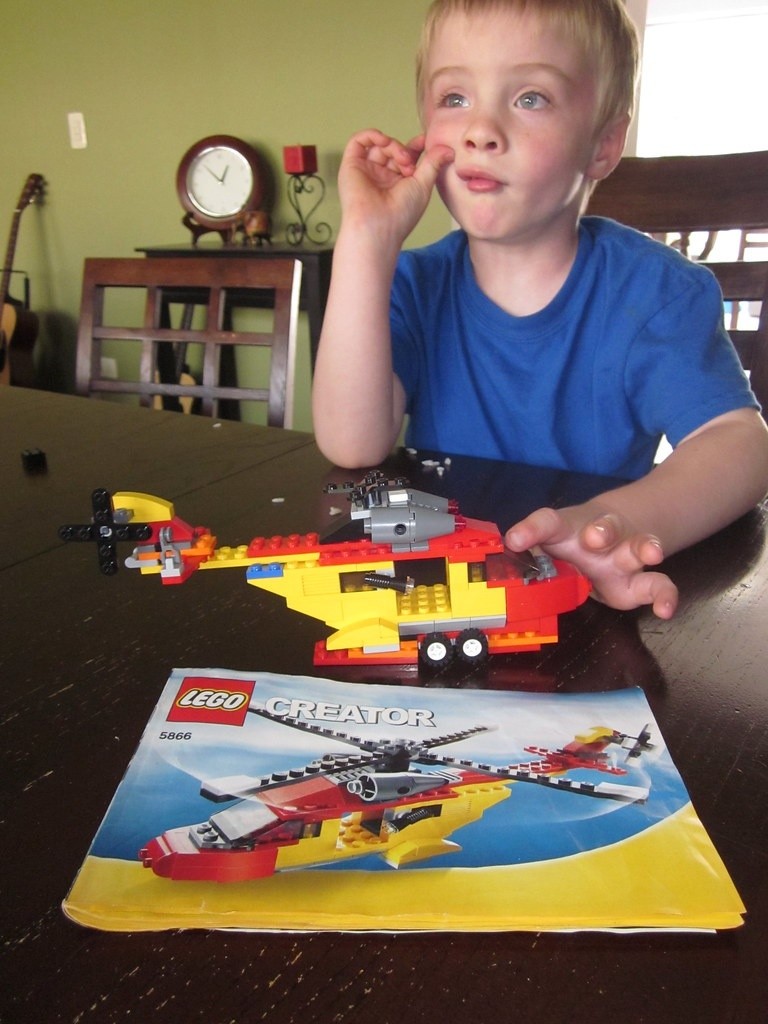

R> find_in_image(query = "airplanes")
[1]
[59,470,596,673]
[138,702,662,885]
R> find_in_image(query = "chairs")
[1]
[584,151,767,429]
[74,255,304,428]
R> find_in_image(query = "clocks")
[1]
[176,132,273,229]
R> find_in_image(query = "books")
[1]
[62,670,747,935]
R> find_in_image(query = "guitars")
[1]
[0,172,44,388]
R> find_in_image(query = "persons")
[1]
[309,0,768,619]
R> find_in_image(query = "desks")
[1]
[135,240,333,413]
[0,390,765,1024]
